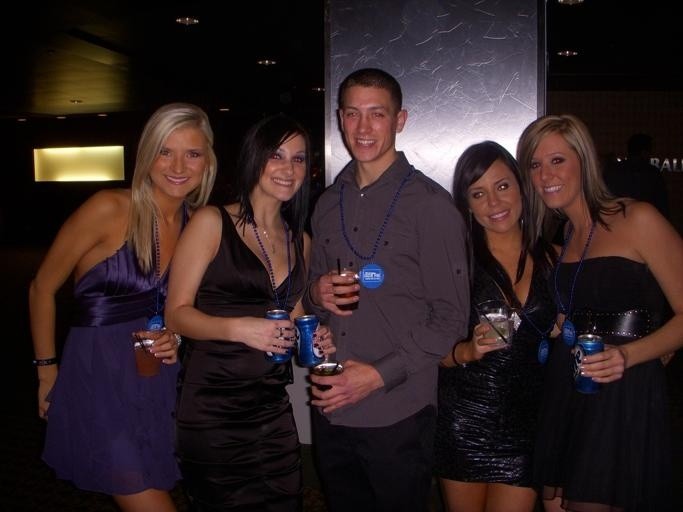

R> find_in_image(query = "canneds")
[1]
[266,310,292,363]
[573,334,604,395]
[294,314,324,368]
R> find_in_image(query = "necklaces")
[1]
[254,213,283,254]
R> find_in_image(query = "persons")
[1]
[301,67,468,511]
[26,104,217,511]
[164,114,311,511]
[517,115,683,511]
[605,131,672,219]
[435,141,559,511]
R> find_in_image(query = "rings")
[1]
[279,328,283,339]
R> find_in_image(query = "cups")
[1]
[333,278,360,311]
[474,299,513,348]
[131,329,162,379]
[312,362,344,392]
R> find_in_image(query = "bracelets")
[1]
[32,357,59,366]
[452,340,466,367]
[162,326,182,347]
